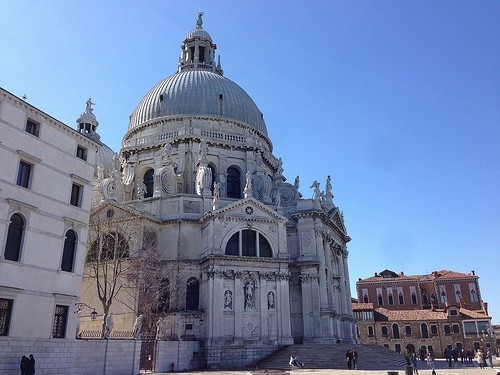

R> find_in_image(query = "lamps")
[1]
[171,361,175,372]
[74,301,98,320]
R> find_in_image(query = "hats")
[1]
[477,349,481,351]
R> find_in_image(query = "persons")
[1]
[155,317,183,341]
[351,349,359,370]
[345,349,353,370]
[225,280,275,312]
[27,354,36,375]
[104,313,114,339]
[81,95,334,217]
[20,355,30,375]
[405,347,494,375]
[132,314,143,337]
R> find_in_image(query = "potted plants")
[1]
[401,349,418,375]
